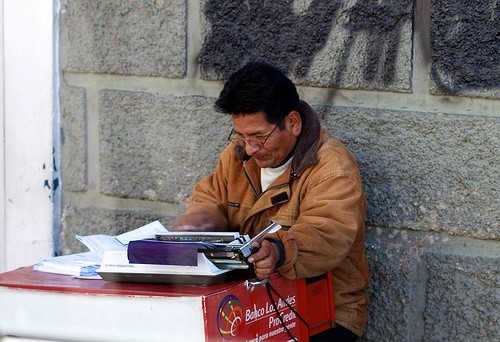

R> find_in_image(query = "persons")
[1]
[171,61,371,342]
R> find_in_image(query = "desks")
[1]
[0,242,336,342]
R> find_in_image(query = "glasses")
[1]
[228,120,283,148]
